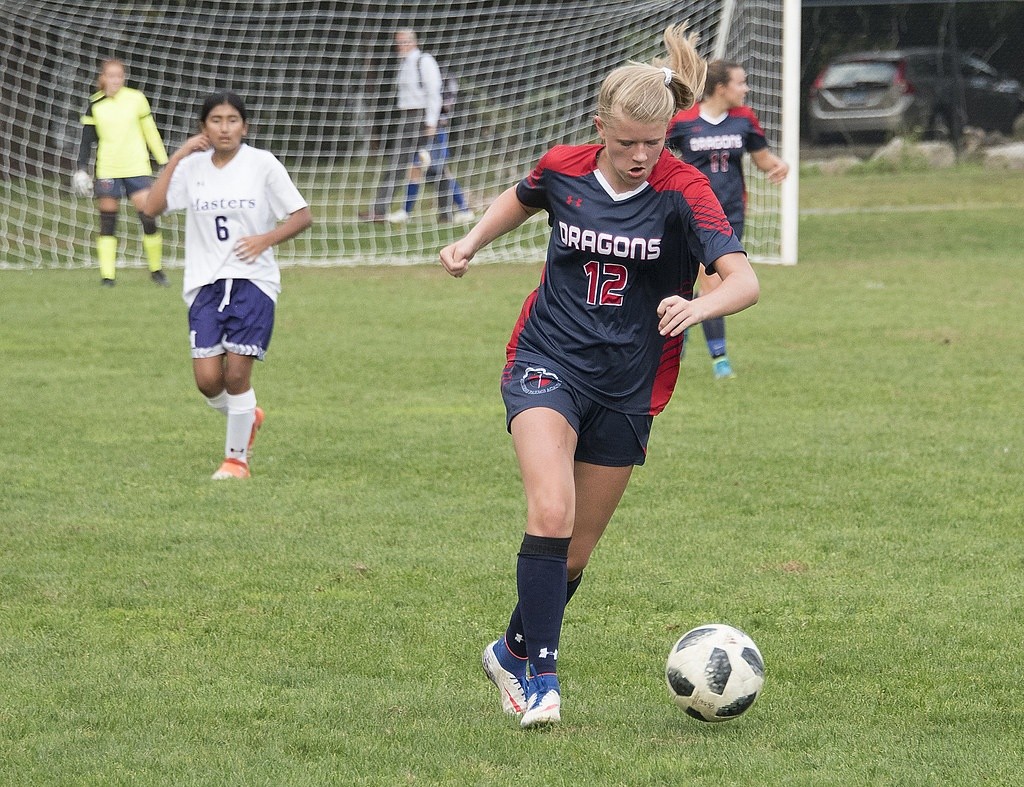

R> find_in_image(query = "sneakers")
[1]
[211,457,254,482]
[479,631,526,718]
[519,663,561,726]
[247,407,267,460]
[713,357,735,377]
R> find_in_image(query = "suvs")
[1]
[807,48,1023,146]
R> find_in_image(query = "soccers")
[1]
[664,622,767,723]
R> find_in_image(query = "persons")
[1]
[144,93,312,479]
[72,60,170,288]
[441,17,759,727]
[355,26,477,224]
[662,59,788,379]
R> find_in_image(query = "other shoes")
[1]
[102,278,114,287]
[357,211,385,223]
[385,210,409,223]
[152,269,169,288]
[453,210,474,223]
[437,214,448,221]
